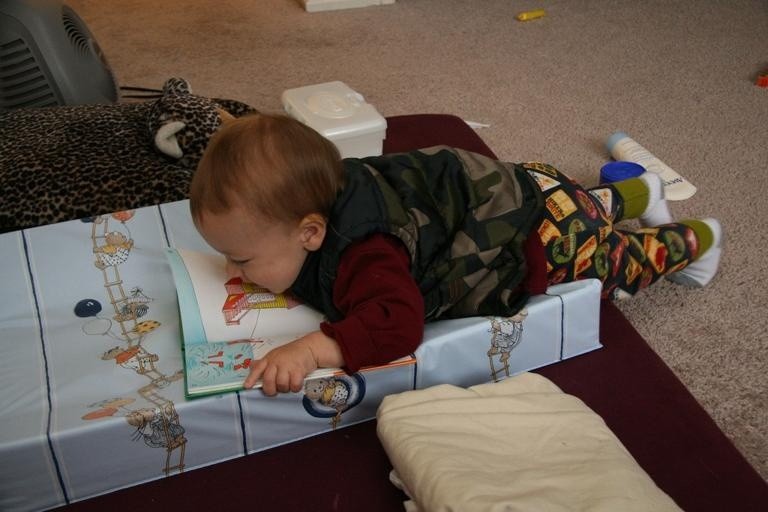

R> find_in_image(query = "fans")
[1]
[0,0,123,111]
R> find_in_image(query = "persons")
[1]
[190,112,724,396]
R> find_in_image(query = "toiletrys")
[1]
[608,132,698,202]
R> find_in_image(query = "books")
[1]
[164,248,417,401]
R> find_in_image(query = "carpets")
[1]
[42,110,765,510]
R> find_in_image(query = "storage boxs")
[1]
[279,78,391,164]
